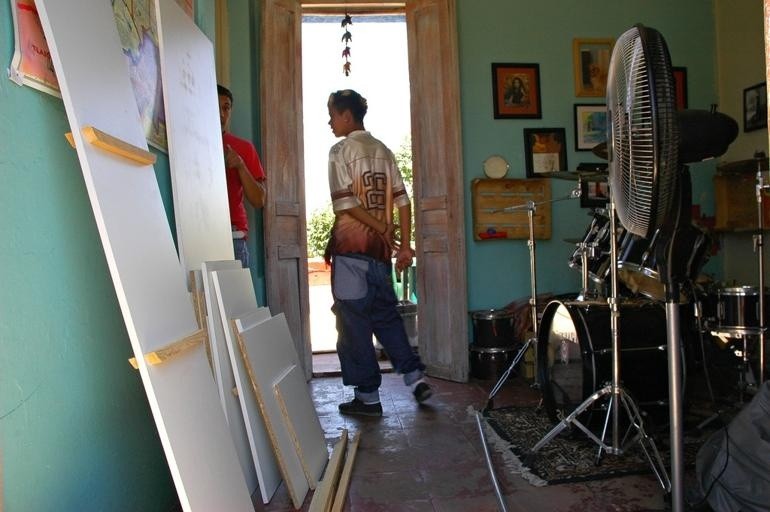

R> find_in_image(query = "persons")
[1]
[506,78,527,105]
[328,89,433,420]
[217,81,265,271]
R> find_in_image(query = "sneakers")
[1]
[338,395,383,418]
[412,379,434,404]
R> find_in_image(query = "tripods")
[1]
[481,176,581,415]
[530,199,672,501]
[697,339,750,431]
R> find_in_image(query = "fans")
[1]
[605,22,738,511]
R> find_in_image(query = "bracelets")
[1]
[381,224,389,237]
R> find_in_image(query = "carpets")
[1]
[465,400,703,487]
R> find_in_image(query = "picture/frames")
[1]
[743,82,767,133]
[490,38,690,179]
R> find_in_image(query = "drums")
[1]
[537,300,686,441]
[472,310,519,346]
[617,207,720,306]
[569,207,627,284]
[688,284,769,339]
[468,343,522,379]
